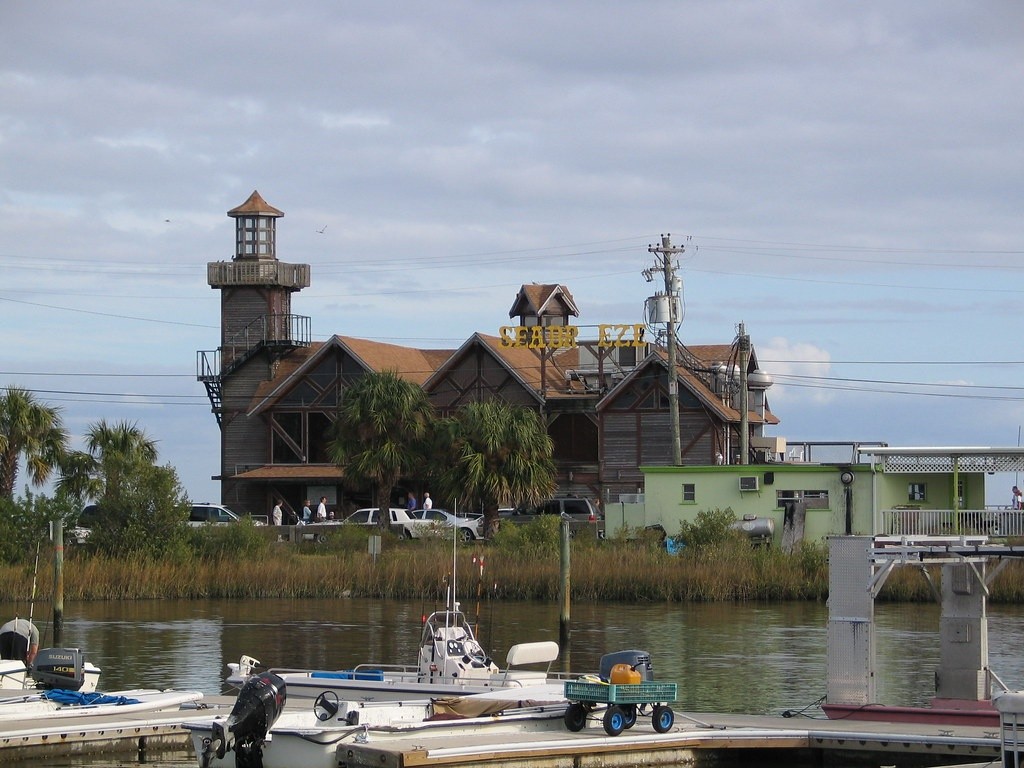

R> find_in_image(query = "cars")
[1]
[297,507,518,548]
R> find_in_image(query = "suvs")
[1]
[476,493,604,541]
[172,502,268,526]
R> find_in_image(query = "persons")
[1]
[303,501,312,524]
[0,619,39,675]
[273,500,283,541]
[1012,487,1022,510]
[568,493,599,512]
[328,512,335,520]
[408,492,417,510]
[423,493,432,509]
[317,497,327,521]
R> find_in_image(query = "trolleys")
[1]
[565,679,726,736]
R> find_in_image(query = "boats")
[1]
[0,520,203,735]
[181,498,668,768]
